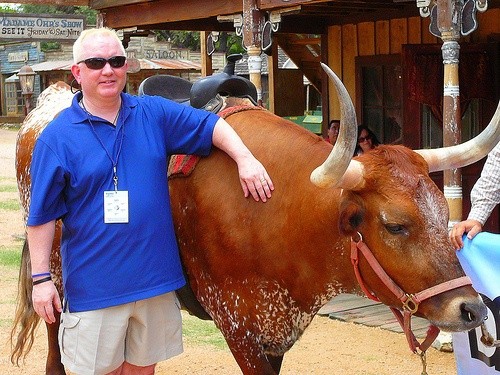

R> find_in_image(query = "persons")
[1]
[356,125,375,153]
[448,140,500,251]
[324,120,340,146]
[25,26,276,375]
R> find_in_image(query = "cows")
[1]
[10,61,500,375]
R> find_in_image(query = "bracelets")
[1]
[33,276,52,285]
[31,272,50,278]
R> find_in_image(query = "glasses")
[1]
[76,56,126,70]
[357,135,370,143]
